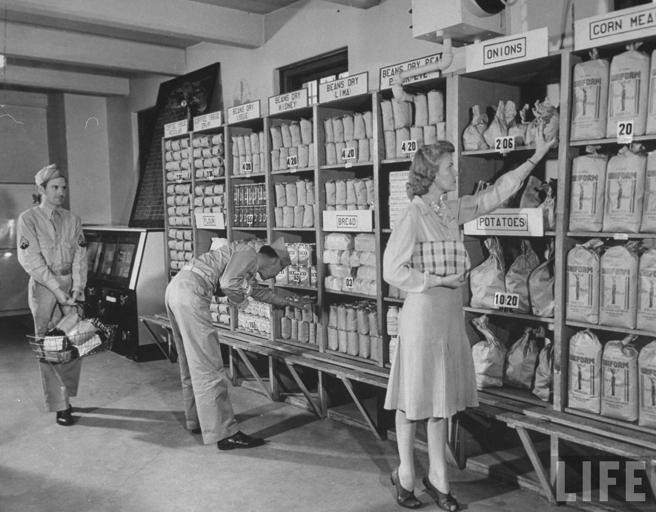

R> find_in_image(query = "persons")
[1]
[380,120,557,512]
[15,162,91,427]
[163,235,315,450]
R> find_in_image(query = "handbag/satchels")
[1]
[403,241,472,282]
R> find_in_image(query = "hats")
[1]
[270,236,291,270]
[34,163,64,186]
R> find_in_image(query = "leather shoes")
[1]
[390,468,422,508]
[57,408,71,425]
[217,431,265,450]
[423,476,458,512]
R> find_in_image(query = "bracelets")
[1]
[527,158,536,167]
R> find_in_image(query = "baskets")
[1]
[25,300,118,369]
